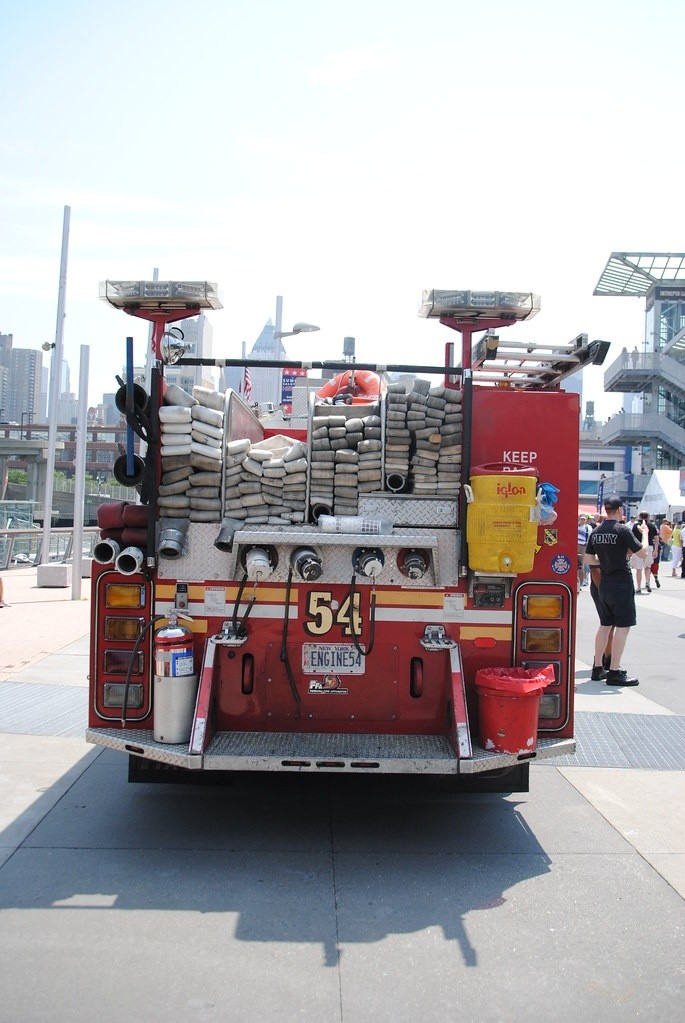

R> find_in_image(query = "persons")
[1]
[607,417,611,421]
[600,473,606,479]
[628,471,634,474]
[640,467,647,475]
[586,496,649,686]
[660,519,685,579]
[577,512,608,594]
[621,408,625,413]
[622,511,662,593]
[649,469,654,475]
[622,347,629,369]
[631,346,639,369]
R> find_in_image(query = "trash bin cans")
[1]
[476,668,543,753]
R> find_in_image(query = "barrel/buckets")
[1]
[474,667,542,754]
[463,462,543,574]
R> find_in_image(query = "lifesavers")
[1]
[319,371,387,407]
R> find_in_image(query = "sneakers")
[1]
[606,670,639,686]
[591,666,609,680]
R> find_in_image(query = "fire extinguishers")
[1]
[152,607,195,678]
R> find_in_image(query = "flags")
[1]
[238,357,252,403]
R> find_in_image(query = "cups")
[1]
[321,516,382,536]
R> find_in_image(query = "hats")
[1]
[639,511,649,520]
[677,520,684,524]
[580,514,586,519]
[585,513,592,519]
[604,497,628,510]
[630,515,636,519]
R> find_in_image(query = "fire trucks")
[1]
[86,281,610,796]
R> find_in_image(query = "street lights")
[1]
[21,413,28,440]
[29,413,36,424]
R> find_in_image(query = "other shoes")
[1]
[655,579,660,588]
[636,590,641,593]
[672,574,678,578]
[594,653,620,670]
[645,584,652,592]
